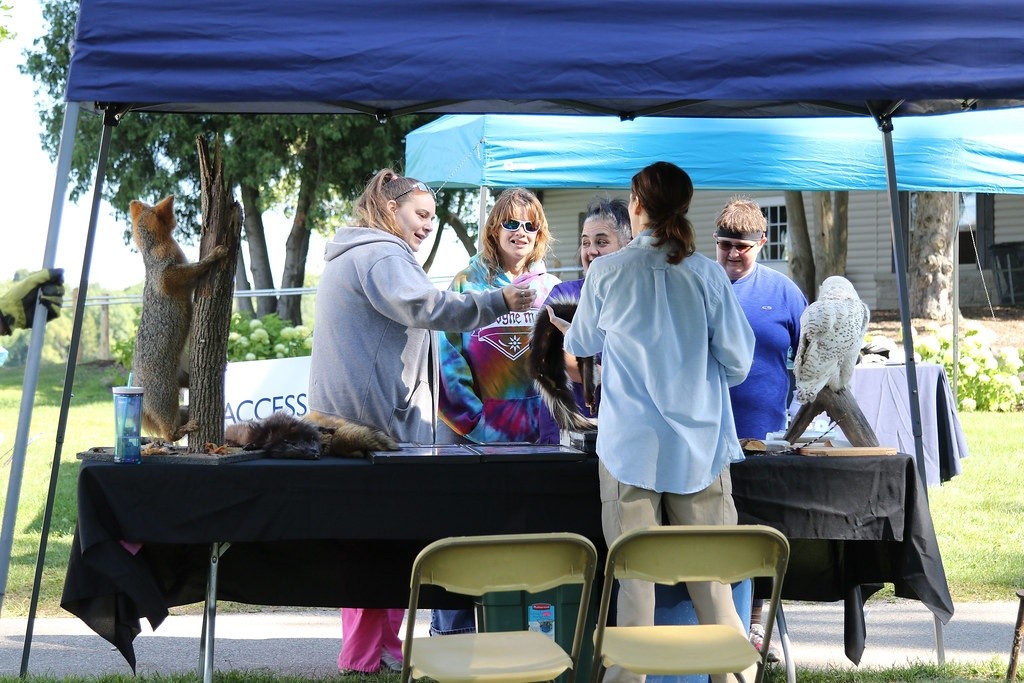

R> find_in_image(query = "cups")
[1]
[113,386,144,464]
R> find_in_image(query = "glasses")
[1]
[500,218,540,234]
[394,182,437,200]
[717,241,760,251]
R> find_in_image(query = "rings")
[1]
[521,296,525,303]
[522,303,525,309]
[520,290,525,297]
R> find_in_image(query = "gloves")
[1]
[0,268,65,336]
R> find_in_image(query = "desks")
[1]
[58,442,954,683]
[786,361,968,488]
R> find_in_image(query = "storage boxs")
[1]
[560,429,598,452]
[471,578,755,683]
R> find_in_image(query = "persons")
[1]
[308,167,538,676]
[540,198,632,444]
[711,195,808,661]
[428,187,562,637]
[0,268,65,336]
[563,162,757,683]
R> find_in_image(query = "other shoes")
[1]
[750,623,781,663]
[377,644,402,675]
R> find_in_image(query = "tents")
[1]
[0,0,1023,683]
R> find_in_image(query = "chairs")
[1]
[400,533,598,683]
[593,525,791,683]
